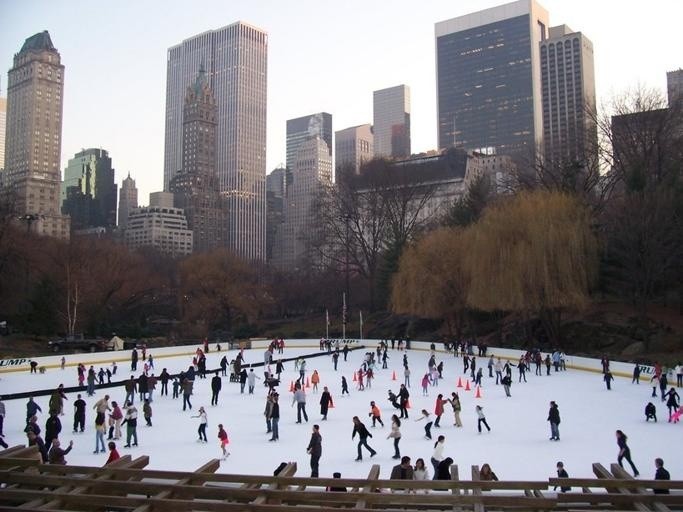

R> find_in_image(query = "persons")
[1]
[88,371,97,395]
[652,458,671,494]
[603,370,613,390]
[192,407,209,443]
[325,472,348,494]
[78,363,85,386]
[144,362,149,375]
[125,375,137,406]
[397,336,403,351]
[544,354,551,376]
[341,375,349,396]
[311,370,320,394]
[291,383,310,423]
[320,386,332,420]
[99,368,106,385]
[236,352,243,370]
[452,340,459,358]
[264,344,273,366]
[412,458,429,480]
[480,463,498,481]
[460,341,466,357]
[357,368,365,391]
[143,399,152,427]
[307,425,323,480]
[230,359,236,382]
[448,392,462,427]
[387,414,401,460]
[39,366,46,373]
[405,335,410,351]
[89,365,95,373]
[160,368,171,396]
[644,402,658,423]
[518,360,528,383]
[535,352,542,376]
[264,394,274,432]
[429,342,435,354]
[368,401,384,427]
[203,336,209,354]
[264,360,272,385]
[216,340,222,351]
[404,365,411,388]
[547,402,562,440]
[402,354,409,368]
[74,394,86,433]
[434,394,448,427]
[479,340,482,357]
[142,343,147,360]
[336,340,340,351]
[427,355,436,370]
[112,360,118,376]
[559,352,567,370]
[29,360,38,373]
[46,409,61,443]
[613,429,639,477]
[249,370,261,395]
[138,372,148,402]
[187,367,196,382]
[327,341,332,353]
[93,394,110,412]
[266,374,278,393]
[495,357,503,384]
[196,348,203,358]
[438,457,452,480]
[664,388,681,424]
[421,374,429,396]
[502,373,513,397]
[131,347,138,370]
[109,399,121,440]
[0,396,6,437]
[193,357,198,368]
[432,435,445,480]
[650,374,658,397]
[674,362,683,387]
[94,411,106,453]
[61,357,66,369]
[219,355,227,377]
[269,396,280,442]
[25,431,47,465]
[432,375,432,379]
[239,369,248,393]
[296,361,307,386]
[388,390,400,409]
[26,396,41,425]
[279,337,284,354]
[487,354,495,378]
[105,442,121,464]
[437,361,443,378]
[379,337,388,351]
[389,456,413,480]
[198,354,208,379]
[217,424,231,461]
[382,350,388,369]
[473,368,482,388]
[104,368,112,385]
[504,360,516,377]
[463,353,470,374]
[148,354,153,368]
[49,439,73,466]
[58,383,68,415]
[377,346,383,363]
[600,353,609,375]
[395,384,410,419]
[331,347,340,371]
[415,409,433,441]
[148,373,157,401]
[431,367,439,387]
[352,416,376,460]
[277,359,284,379]
[631,363,641,384]
[49,389,63,417]
[343,345,350,360]
[274,336,279,350]
[124,402,138,447]
[363,350,375,367]
[525,351,531,370]
[211,371,221,405]
[659,374,668,400]
[173,377,180,399]
[653,361,661,381]
[552,350,561,372]
[551,462,574,493]
[402,354,410,369]
[475,405,491,432]
[295,357,305,369]
[390,335,396,348]
[24,415,40,445]
[365,368,373,388]
[320,337,325,351]
[180,378,193,411]
[482,342,487,357]
[471,357,477,382]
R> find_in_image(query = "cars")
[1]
[48,332,107,353]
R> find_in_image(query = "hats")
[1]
[199,407,205,411]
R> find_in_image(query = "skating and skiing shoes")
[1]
[550,437,555,440]
[555,438,560,441]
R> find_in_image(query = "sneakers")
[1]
[296,422,302,424]
[94,451,98,454]
[132,443,137,447]
[404,417,408,419]
[355,458,363,462]
[276,437,278,440]
[424,436,428,438]
[196,439,202,441]
[435,425,440,427]
[269,439,276,442]
[322,419,327,421]
[100,449,105,452]
[124,444,129,448]
[457,425,462,427]
[219,458,226,461]
[392,456,396,458]
[79,430,84,433]
[399,417,404,419]
[371,425,376,428]
[394,456,400,459]
[202,441,207,443]
[454,424,457,426]
[370,452,376,457]
[225,452,230,457]
[427,438,432,440]
[72,430,77,433]
[382,424,384,428]
[306,418,308,422]
